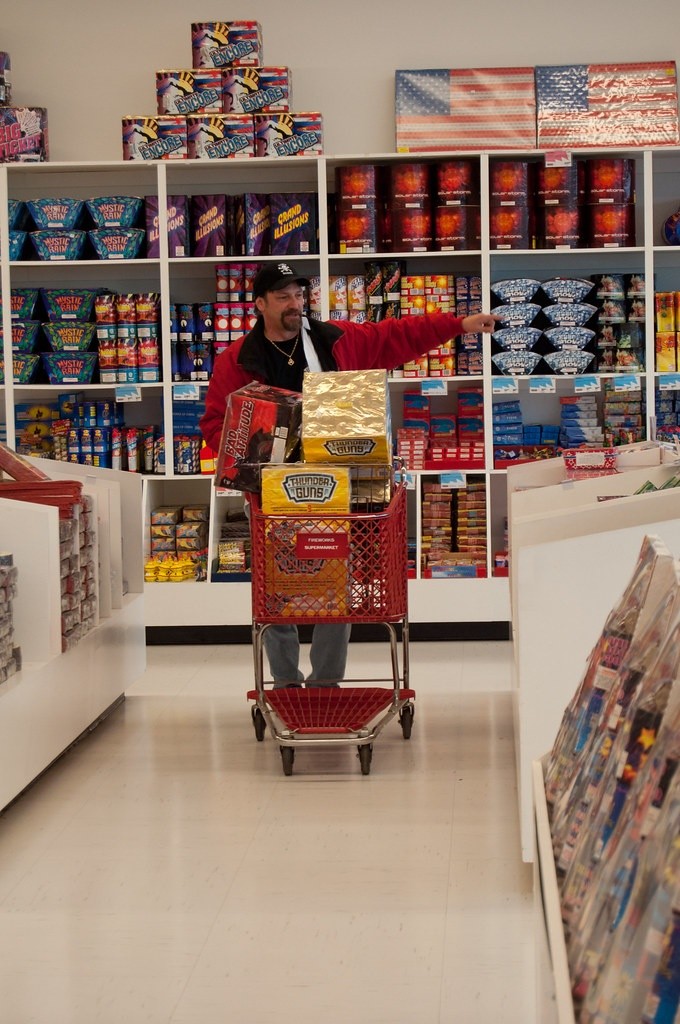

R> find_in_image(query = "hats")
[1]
[253,262,310,293]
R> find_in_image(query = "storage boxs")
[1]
[213,369,394,613]
[536,59,679,148]
[397,386,486,470]
[589,273,680,372]
[255,112,323,158]
[143,192,316,262]
[145,505,209,574]
[192,20,262,67]
[394,66,536,152]
[494,378,648,445]
[422,481,488,577]
[401,274,484,377]
[156,68,221,114]
[0,106,51,163]
[16,392,124,465]
[337,160,634,250]
[121,114,188,159]
[188,114,255,157]
[223,67,294,114]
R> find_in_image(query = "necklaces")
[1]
[265,334,299,366]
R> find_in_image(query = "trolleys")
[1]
[247,452,416,777]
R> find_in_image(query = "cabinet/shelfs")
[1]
[0,145,680,628]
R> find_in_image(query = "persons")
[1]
[199,260,504,689]
